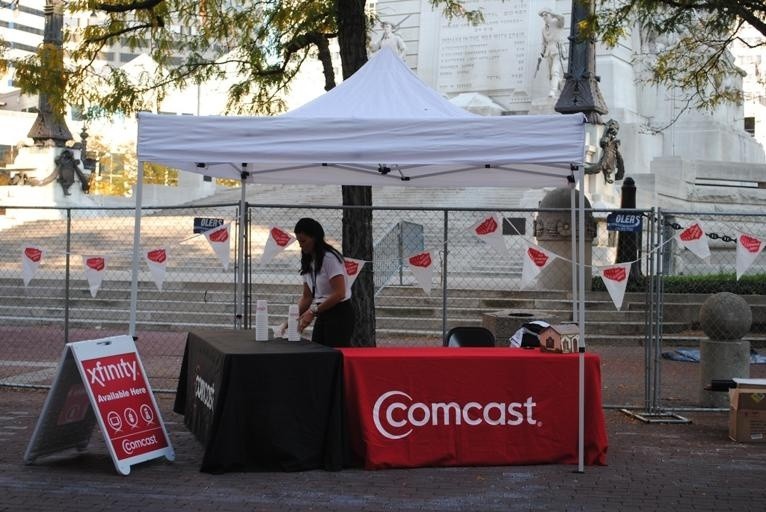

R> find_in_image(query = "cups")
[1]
[287,303,300,342]
[255,299,269,341]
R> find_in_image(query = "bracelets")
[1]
[309,305,321,317]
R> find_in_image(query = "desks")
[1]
[334,346,610,470]
[172,329,356,474]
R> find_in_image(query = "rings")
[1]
[303,324,307,328]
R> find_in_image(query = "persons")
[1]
[273,216,356,348]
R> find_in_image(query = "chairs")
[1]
[442,326,496,347]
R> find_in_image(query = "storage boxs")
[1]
[727,388,766,443]
